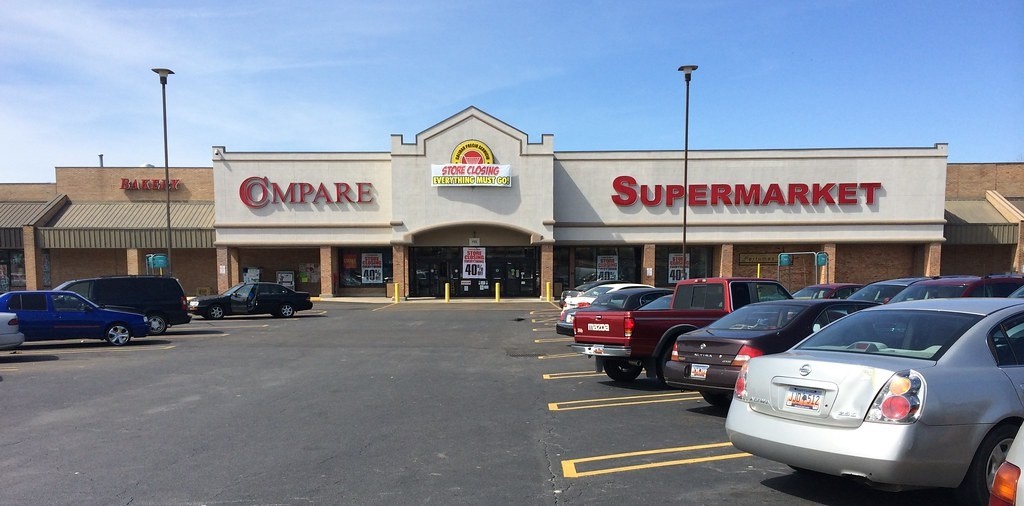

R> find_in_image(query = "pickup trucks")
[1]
[570,277,794,390]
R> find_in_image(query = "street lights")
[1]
[151,68,175,277]
[678,64,698,280]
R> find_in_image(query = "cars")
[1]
[664,299,883,408]
[0,313,24,351]
[555,288,675,337]
[560,283,656,322]
[988,422,1023,506]
[846,277,931,304]
[0,291,148,346]
[188,282,313,320]
[792,283,866,300]
[725,297,1024,506]
[559,280,633,308]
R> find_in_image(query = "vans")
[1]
[52,275,192,336]
[873,275,1024,335]
